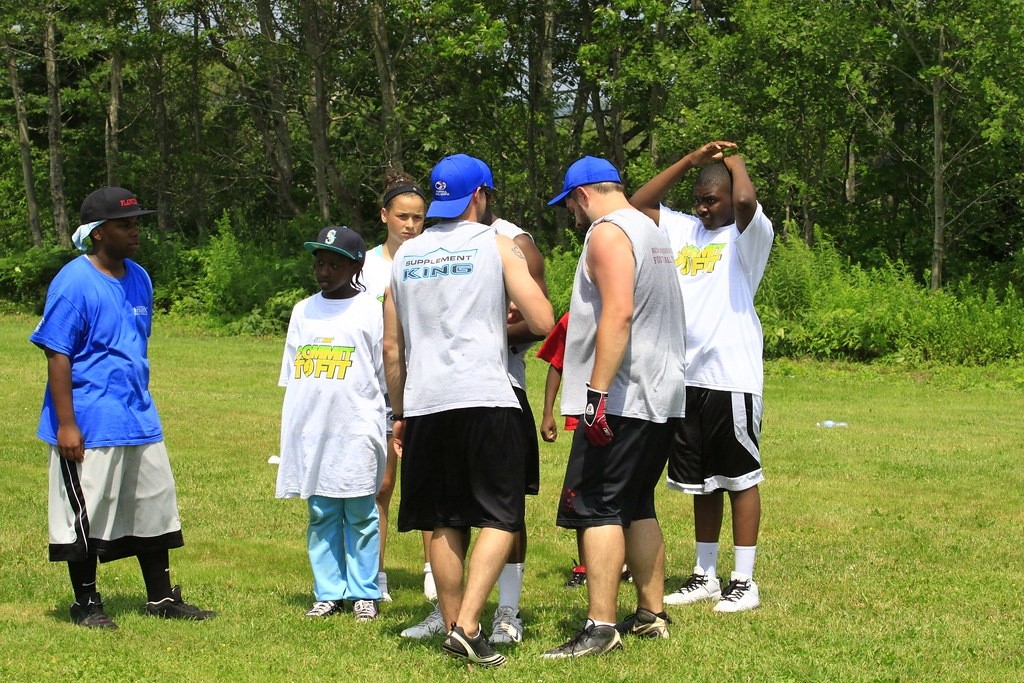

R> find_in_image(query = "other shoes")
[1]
[423,587,438,601]
[377,591,392,602]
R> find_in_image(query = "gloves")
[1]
[584,382,613,446]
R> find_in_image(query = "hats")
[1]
[80,186,158,224]
[547,156,622,205]
[426,154,481,217]
[472,158,494,189]
[304,225,365,266]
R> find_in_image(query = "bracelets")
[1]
[390,414,405,422]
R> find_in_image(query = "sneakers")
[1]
[354,599,379,622]
[564,564,587,587]
[619,564,635,582]
[487,605,524,644]
[146,584,215,620]
[663,565,721,604]
[401,604,448,639]
[306,598,344,617]
[69,592,118,631]
[443,621,506,668]
[544,618,623,660]
[615,608,673,639]
[713,571,758,611]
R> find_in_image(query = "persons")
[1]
[535,155,688,658]
[385,153,554,665]
[31,187,220,631]
[400,157,548,642]
[535,313,634,586]
[358,169,442,600]
[272,226,389,622]
[629,138,776,610]
